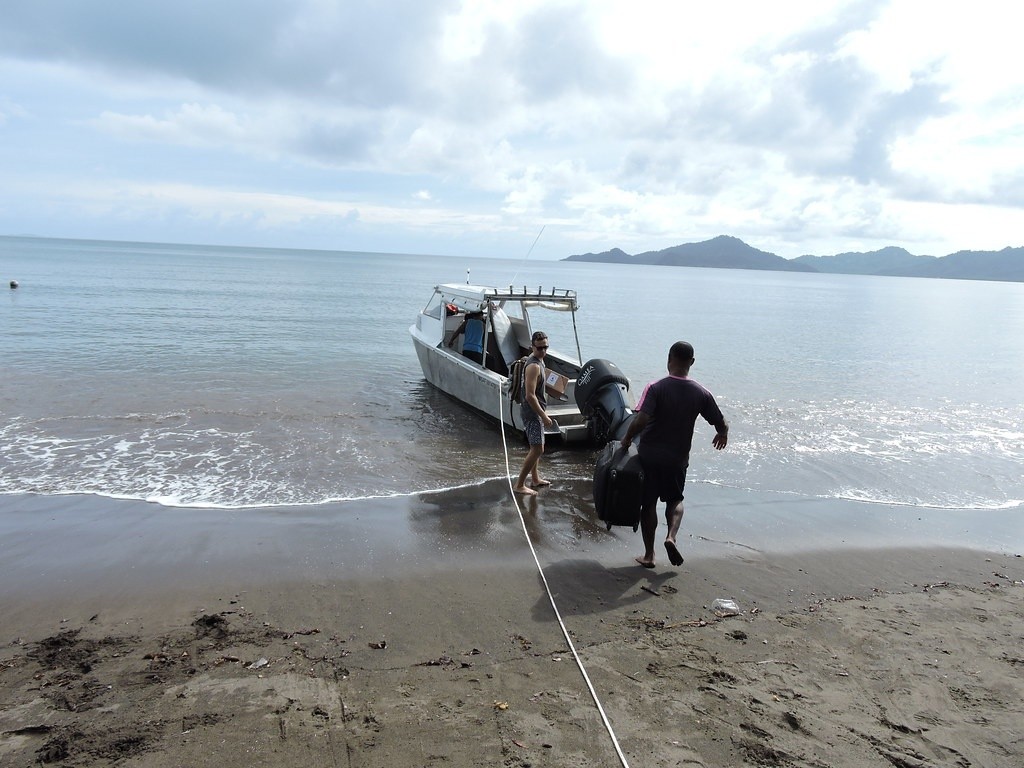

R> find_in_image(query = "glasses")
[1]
[534,345,549,351]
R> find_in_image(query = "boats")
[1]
[406,268,637,448]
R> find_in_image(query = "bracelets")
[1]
[719,434,727,438]
[624,439,631,446]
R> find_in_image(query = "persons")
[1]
[620,340,729,567]
[511,331,554,496]
[448,310,485,366]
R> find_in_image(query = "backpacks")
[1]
[507,356,529,404]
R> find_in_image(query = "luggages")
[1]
[593,441,644,532]
